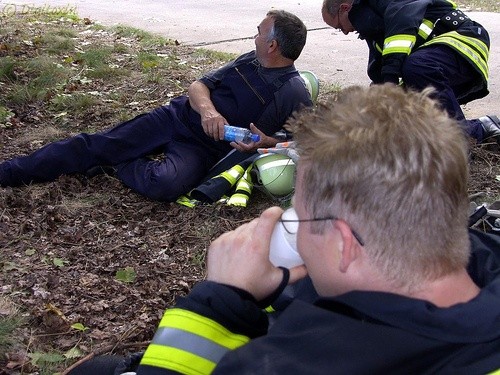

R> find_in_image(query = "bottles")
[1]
[224,125,260,144]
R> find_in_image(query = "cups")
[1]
[269,207,304,269]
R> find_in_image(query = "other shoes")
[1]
[480,116,500,141]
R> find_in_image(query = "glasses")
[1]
[335,8,343,32]
[279,206,365,249]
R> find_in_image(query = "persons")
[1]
[322,0,500,147]
[0,10,314,204]
[65,86,500,375]
